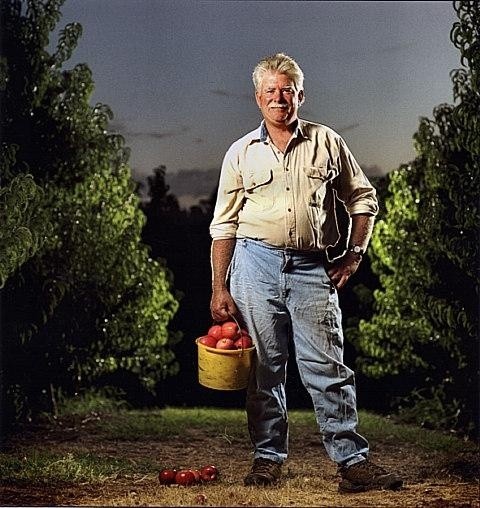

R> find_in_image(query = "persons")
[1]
[208,52,404,495]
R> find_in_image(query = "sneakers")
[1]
[338,460,403,492]
[245,457,281,484]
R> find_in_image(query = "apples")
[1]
[195,495,206,505]
[201,465,218,482]
[208,325,222,340]
[237,328,248,338]
[159,470,176,482]
[200,336,217,349]
[222,322,240,337]
[191,470,200,481]
[217,339,235,350]
[236,337,252,349]
[174,472,195,485]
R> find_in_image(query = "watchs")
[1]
[347,243,367,257]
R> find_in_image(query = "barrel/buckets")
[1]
[195,312,256,390]
